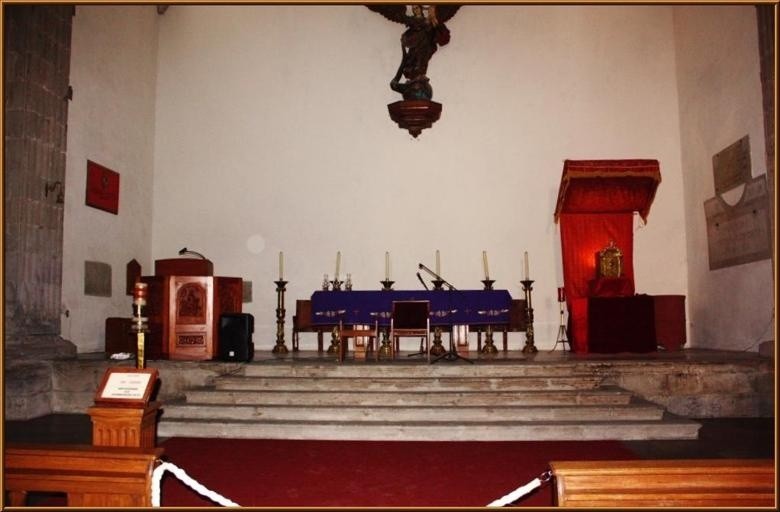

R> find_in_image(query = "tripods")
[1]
[418,263,473,363]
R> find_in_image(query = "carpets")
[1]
[148,433,645,507]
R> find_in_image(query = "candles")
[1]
[483,250,490,281]
[435,250,440,277]
[334,251,341,280]
[278,252,284,280]
[524,251,530,281]
[385,251,389,281]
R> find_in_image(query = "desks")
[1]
[292,289,529,353]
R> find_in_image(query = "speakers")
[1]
[217,310,256,361]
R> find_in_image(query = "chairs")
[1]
[389,300,432,362]
